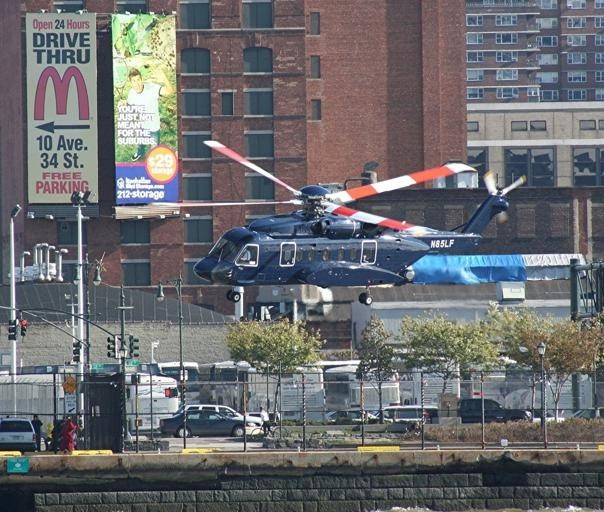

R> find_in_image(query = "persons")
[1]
[259,406,274,436]
[117,68,172,162]
[57,415,78,455]
[404,421,419,435]
[29,412,44,452]
[76,410,84,437]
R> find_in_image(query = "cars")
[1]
[574,407,604,420]
[316,409,379,425]
[179,404,281,428]
[157,409,249,439]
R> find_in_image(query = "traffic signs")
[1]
[126,361,140,366]
[92,363,103,369]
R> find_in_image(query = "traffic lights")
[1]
[130,334,139,359]
[164,389,171,397]
[106,336,115,358]
[20,319,27,337]
[7,319,16,341]
[72,340,79,362]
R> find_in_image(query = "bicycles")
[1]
[250,420,289,443]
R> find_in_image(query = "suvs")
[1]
[457,397,532,423]
[0,415,38,453]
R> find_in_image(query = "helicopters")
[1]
[191,138,532,313]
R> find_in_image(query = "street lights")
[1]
[9,202,22,416]
[118,339,127,452]
[67,188,96,450]
[15,242,71,284]
[155,269,189,449]
[78,256,102,450]
[536,338,551,444]
[60,290,80,365]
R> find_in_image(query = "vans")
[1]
[374,404,440,424]
[123,371,182,438]
